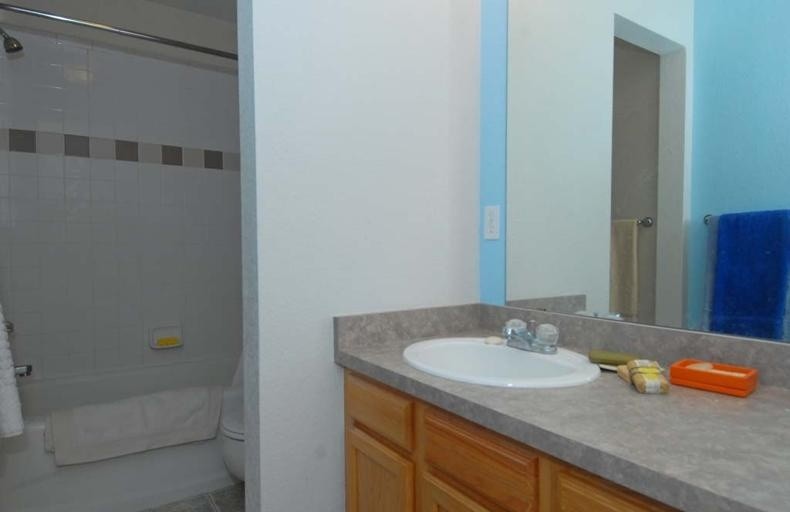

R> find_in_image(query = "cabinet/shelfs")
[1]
[341,366,680,512]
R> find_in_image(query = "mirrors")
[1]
[504,0,788,347]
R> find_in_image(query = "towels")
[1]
[711,209,788,344]
[610,215,642,324]
[43,384,227,467]
[0,301,28,441]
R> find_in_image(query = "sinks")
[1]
[403,334,602,389]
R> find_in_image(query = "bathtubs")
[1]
[1,358,240,511]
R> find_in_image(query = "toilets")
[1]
[220,405,246,480]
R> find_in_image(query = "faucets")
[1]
[602,312,641,323]
[501,327,534,345]
[11,360,34,379]
